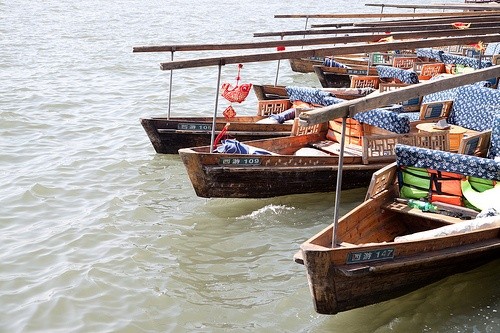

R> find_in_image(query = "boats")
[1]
[131,3,500,154]
[178,73,499,200]
[293,114,500,315]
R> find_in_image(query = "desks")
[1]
[415,123,467,151]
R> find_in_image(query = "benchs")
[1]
[378,142,499,228]
[377,43,500,93]
[307,95,411,156]
